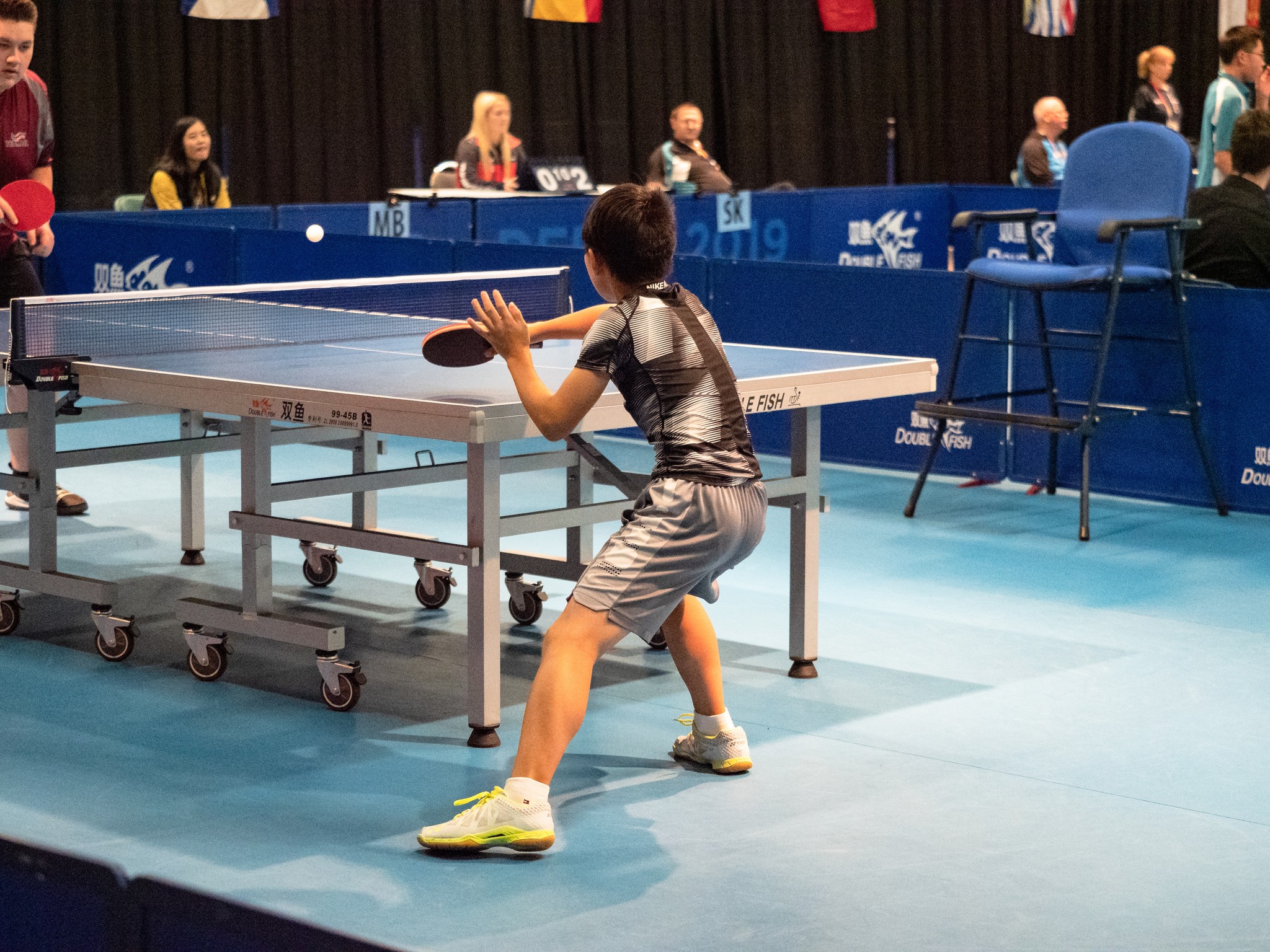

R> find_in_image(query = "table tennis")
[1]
[306,224,324,242]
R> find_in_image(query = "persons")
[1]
[419,184,766,853]
[1192,26,1270,187]
[0,0,88,516]
[1178,110,1270,288]
[141,117,232,210]
[454,90,541,191]
[1126,47,1181,135]
[1017,96,1070,187]
[646,104,798,193]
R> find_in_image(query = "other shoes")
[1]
[673,713,752,774]
[4,484,87,514]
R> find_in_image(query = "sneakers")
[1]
[417,786,556,852]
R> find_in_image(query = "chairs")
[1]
[114,193,146,212]
[429,159,464,189]
[901,118,1232,541]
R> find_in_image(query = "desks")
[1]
[386,181,619,200]
[0,263,942,749]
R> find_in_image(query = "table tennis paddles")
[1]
[422,323,543,368]
[0,180,55,232]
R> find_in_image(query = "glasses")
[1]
[1238,50,1265,62]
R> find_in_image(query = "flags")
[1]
[1218,0,1260,41]
[522,0,602,23]
[1023,0,1078,37]
[817,0,877,32]
[180,0,279,20]
